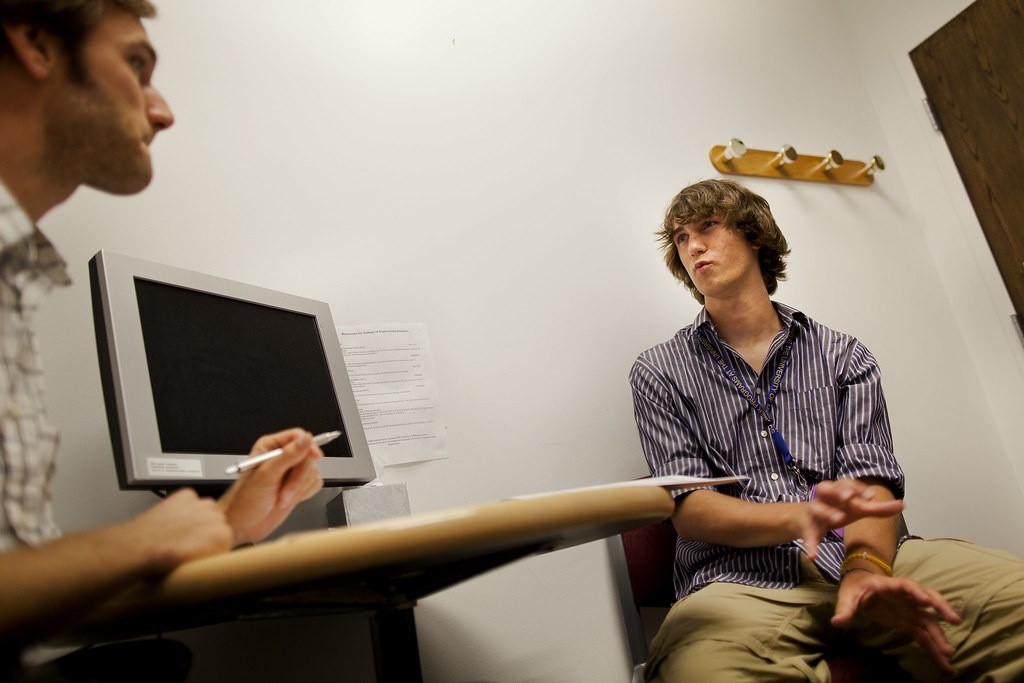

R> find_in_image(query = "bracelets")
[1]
[839,552,893,580]
[840,568,877,579]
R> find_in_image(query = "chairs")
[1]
[607,480,894,683]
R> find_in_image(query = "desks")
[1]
[36,480,680,683]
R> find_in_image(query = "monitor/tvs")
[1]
[87,248,376,491]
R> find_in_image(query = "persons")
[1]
[624,176,1024,683]
[1,0,327,683]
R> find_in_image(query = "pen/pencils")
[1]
[225,430,343,474]
